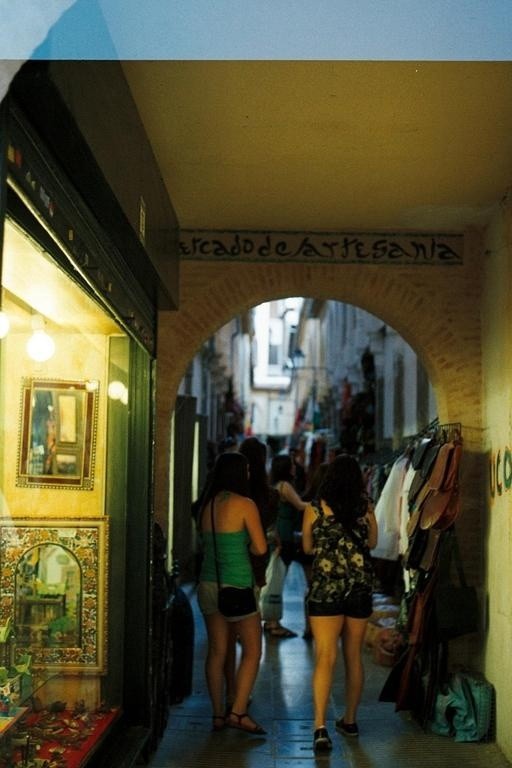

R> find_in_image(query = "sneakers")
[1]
[336,718,359,736]
[315,726,333,748]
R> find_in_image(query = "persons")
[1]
[190,433,330,736]
[300,453,379,752]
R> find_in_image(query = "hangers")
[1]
[394,422,464,491]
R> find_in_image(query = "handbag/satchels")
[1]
[404,588,482,647]
[402,440,462,572]
[218,586,257,617]
[379,658,493,742]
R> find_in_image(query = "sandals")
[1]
[264,620,296,638]
[213,695,266,734]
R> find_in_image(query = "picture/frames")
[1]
[0,516,110,678]
[12,377,99,490]
